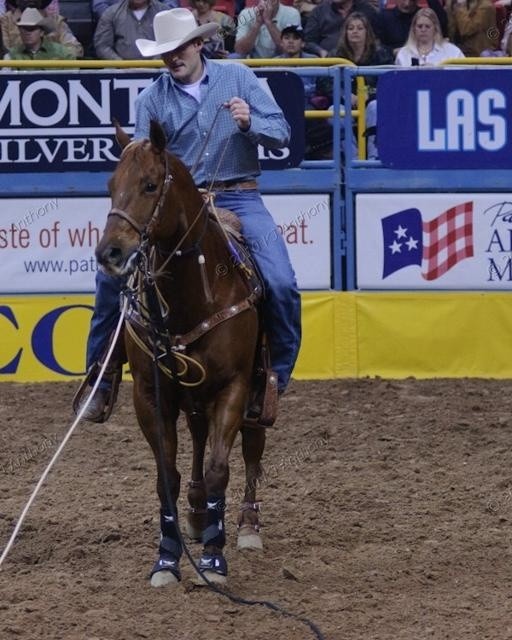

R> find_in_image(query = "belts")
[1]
[199,180,258,192]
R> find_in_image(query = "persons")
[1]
[74,10,303,423]
[0,0,512,161]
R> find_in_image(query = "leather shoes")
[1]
[76,371,114,420]
[245,379,280,420]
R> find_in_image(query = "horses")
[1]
[92,118,270,589]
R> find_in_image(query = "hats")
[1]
[134,7,220,58]
[12,8,55,32]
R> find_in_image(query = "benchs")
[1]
[57,1,118,61]
